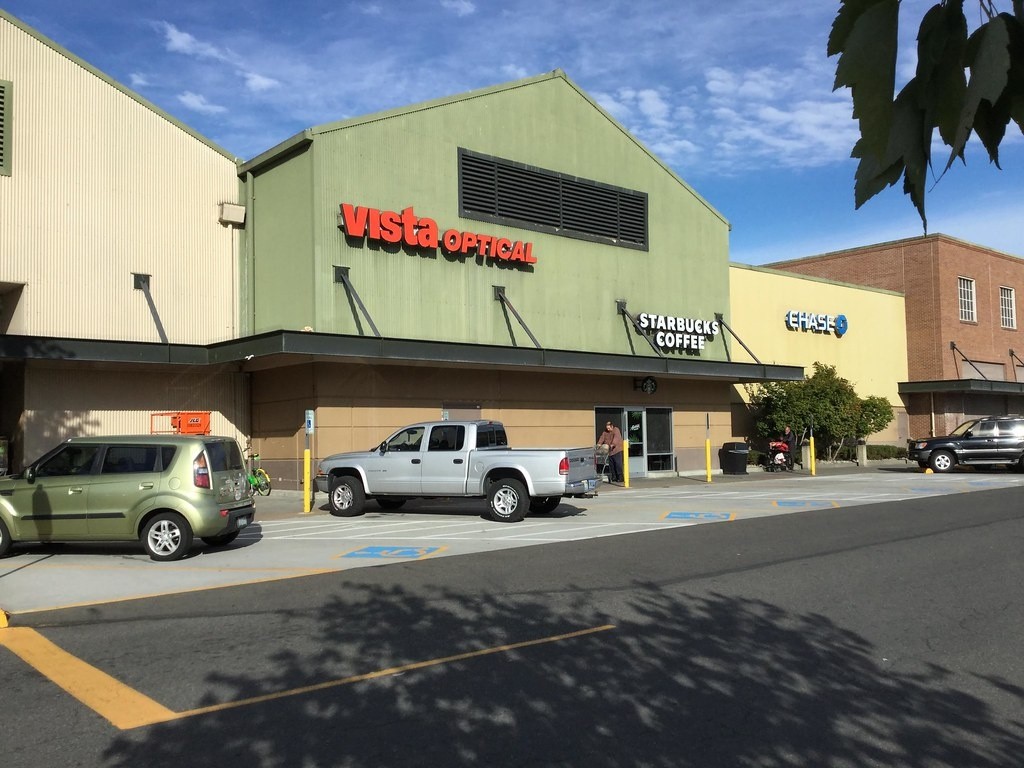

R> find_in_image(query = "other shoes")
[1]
[612,477,616,481]
[616,478,623,482]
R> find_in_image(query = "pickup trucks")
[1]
[313,420,603,523]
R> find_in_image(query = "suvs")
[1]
[908,414,1024,474]
[1,433,255,560]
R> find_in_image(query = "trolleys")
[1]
[595,444,615,484]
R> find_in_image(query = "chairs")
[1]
[117,457,136,472]
[79,452,110,474]
[438,440,449,449]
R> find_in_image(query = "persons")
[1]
[596,422,624,482]
[780,426,796,470]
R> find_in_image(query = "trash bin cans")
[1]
[721,442,749,475]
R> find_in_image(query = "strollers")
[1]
[764,440,791,472]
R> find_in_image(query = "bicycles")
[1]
[243,447,272,496]
